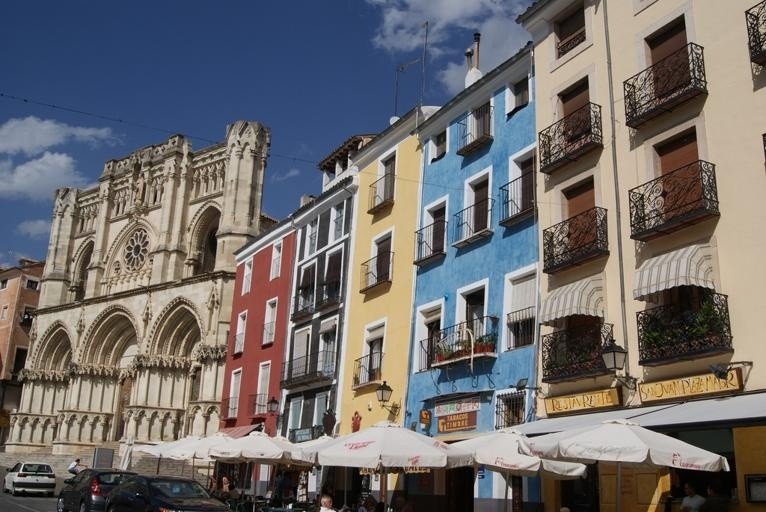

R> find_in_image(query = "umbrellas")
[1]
[516,417,730,512]
[120,435,133,475]
[299,418,452,512]
[134,430,334,511]
[442,427,588,511]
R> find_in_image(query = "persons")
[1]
[67,458,87,474]
[358,494,377,511]
[679,481,706,511]
[700,484,731,511]
[394,496,414,512]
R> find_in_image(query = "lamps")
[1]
[375,381,401,416]
[266,396,281,417]
[601,338,639,390]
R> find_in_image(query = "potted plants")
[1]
[434,333,497,363]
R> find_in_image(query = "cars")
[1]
[55,467,139,512]
[2,460,58,497]
[102,472,231,511]
